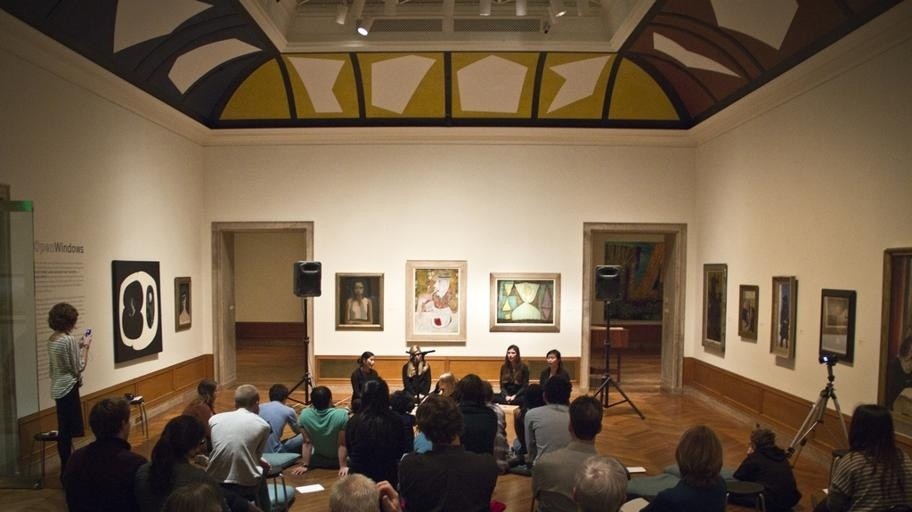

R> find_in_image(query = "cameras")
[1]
[819,352,839,365]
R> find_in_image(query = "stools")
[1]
[28,431,77,482]
[127,396,149,438]
[723,482,765,511]
[259,452,302,512]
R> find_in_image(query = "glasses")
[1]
[200,440,205,446]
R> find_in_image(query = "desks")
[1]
[590,326,626,382]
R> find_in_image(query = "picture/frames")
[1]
[770,276,796,359]
[335,273,384,331]
[702,264,727,353]
[175,277,192,332]
[738,284,759,340]
[405,260,467,343]
[819,289,856,363]
[490,272,561,332]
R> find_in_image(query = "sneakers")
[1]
[506,451,524,467]
[506,464,531,477]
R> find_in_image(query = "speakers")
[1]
[293,260,322,297]
[595,264,624,302]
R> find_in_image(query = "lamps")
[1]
[550,0,567,17]
[350,0,365,18]
[358,16,375,36]
[515,0,527,16]
[336,0,349,24]
[480,0,491,16]
[576,0,589,16]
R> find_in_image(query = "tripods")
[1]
[586,301,646,419]
[785,381,850,468]
[284,298,336,408]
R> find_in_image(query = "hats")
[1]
[750,428,778,449]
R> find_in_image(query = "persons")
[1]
[533,395,605,511]
[637,425,728,511]
[572,455,628,512]
[886,333,912,409]
[351,351,381,414]
[344,280,373,325]
[178,292,190,326]
[290,386,350,477]
[48,302,92,485]
[328,474,402,512]
[727,424,803,511]
[257,383,304,455]
[345,378,413,489]
[204,385,273,512]
[161,482,220,512]
[538,348,571,396]
[188,379,218,458]
[397,394,497,512]
[490,345,532,409]
[389,372,510,475]
[399,346,432,403]
[136,415,230,511]
[814,404,912,512]
[63,396,148,512]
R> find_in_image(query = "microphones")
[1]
[425,350,436,353]
